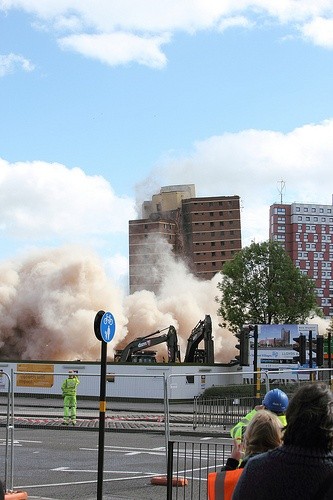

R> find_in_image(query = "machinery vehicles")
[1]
[105,325,182,382]
[183,315,215,384]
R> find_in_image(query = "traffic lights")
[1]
[235,328,249,365]
[293,336,307,367]
[312,335,323,367]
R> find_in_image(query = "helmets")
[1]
[263,388,288,412]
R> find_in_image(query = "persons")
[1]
[221,411,283,472]
[230,388,289,467]
[232,384,333,500]
[61,370,80,426]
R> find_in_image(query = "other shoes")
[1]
[61,421,69,425]
[72,421,76,425]
